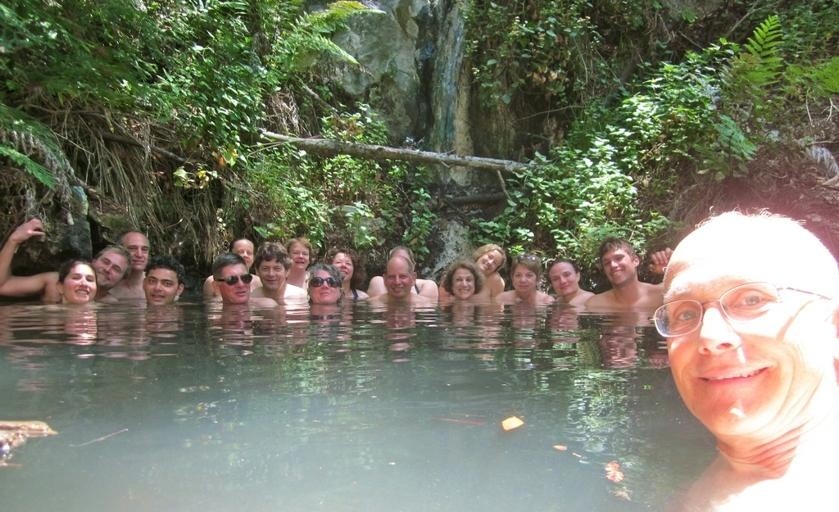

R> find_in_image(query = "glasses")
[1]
[214,274,253,286]
[645,281,836,338]
[309,277,341,288]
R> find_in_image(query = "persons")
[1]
[47,255,113,310]
[583,234,662,310]
[493,252,555,306]
[104,227,154,299]
[0,216,132,306]
[657,210,839,511]
[203,237,506,311]
[140,253,187,305]
[547,257,596,307]
